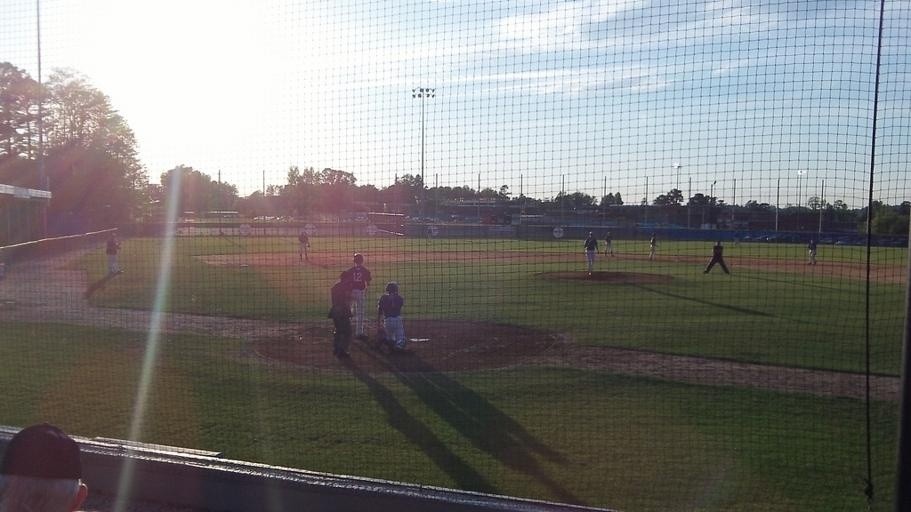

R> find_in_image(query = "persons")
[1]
[703,241,729,274]
[298,231,311,262]
[106,232,121,273]
[808,239,818,265]
[0,422,88,512]
[378,282,407,350]
[328,271,355,357]
[346,254,372,339]
[605,232,614,256]
[649,232,658,261]
[584,232,600,275]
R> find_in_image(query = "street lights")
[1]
[796,169,806,206]
[671,160,682,204]
[411,85,436,215]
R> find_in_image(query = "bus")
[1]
[184,210,242,227]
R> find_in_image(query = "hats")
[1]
[1,422,89,481]
